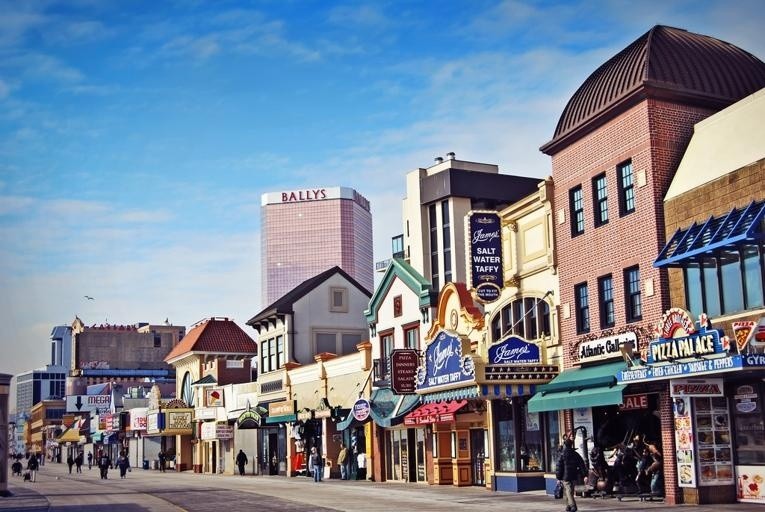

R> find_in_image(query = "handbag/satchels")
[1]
[555,485,564,499]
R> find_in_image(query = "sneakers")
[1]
[566,505,577,512]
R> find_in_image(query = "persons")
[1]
[357,449,367,479]
[158,450,165,472]
[115,451,130,478]
[337,444,349,480]
[311,447,322,482]
[556,439,589,512]
[11,450,113,482]
[236,449,248,476]
